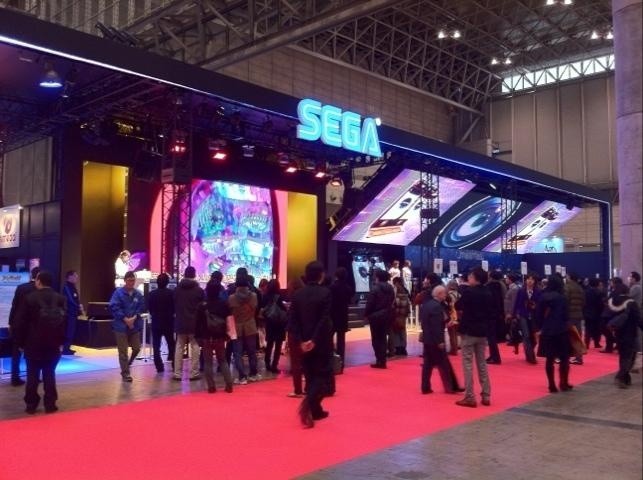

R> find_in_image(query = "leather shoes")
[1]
[481,399,491,407]
[455,399,478,408]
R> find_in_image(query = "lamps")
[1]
[38,52,63,88]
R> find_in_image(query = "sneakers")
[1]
[313,410,329,421]
[299,401,315,428]
[121,364,281,394]
[289,390,302,397]
[616,375,631,389]
[569,357,583,364]
[11,361,633,419]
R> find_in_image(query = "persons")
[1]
[289,260,336,427]
[13,271,64,418]
[113,250,130,290]
[7,267,46,386]
[61,268,78,355]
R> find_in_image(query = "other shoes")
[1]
[26,407,35,415]
[486,357,500,365]
[422,388,435,395]
[445,386,465,393]
[548,383,560,393]
[45,406,59,414]
[12,378,25,387]
[371,362,388,370]
[560,381,573,391]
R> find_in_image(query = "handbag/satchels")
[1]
[227,315,238,342]
[260,294,286,329]
[203,302,228,337]
[606,312,629,333]
[568,325,586,358]
